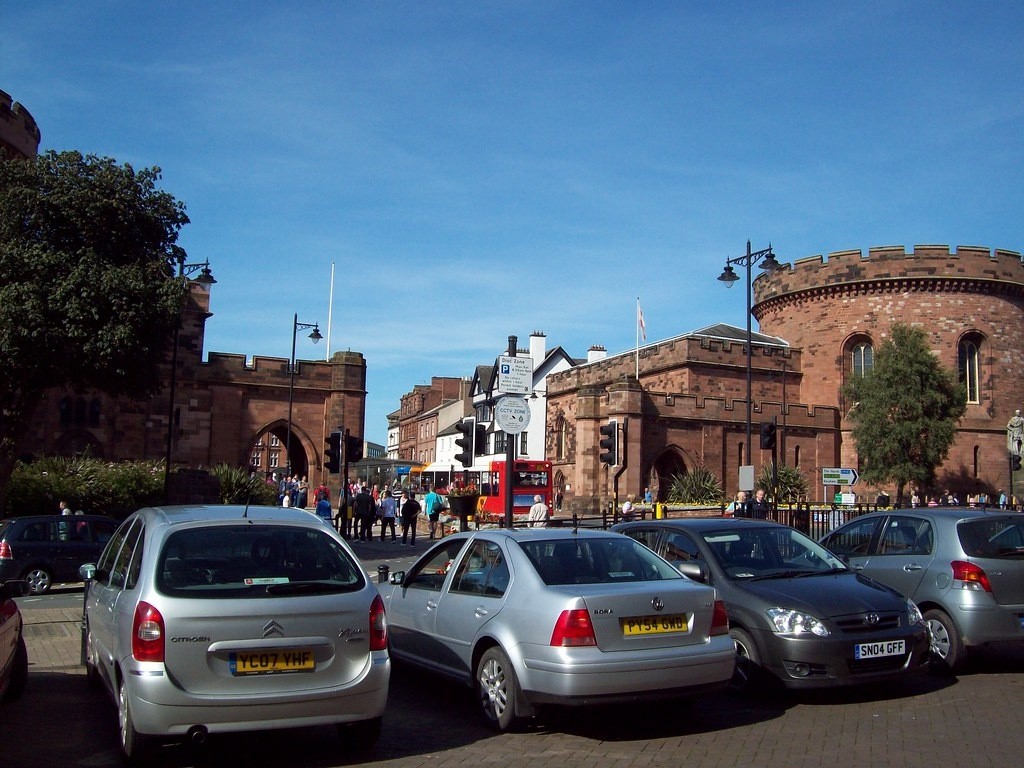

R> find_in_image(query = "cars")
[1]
[80,505,392,768]
[0,580,30,706]
[0,515,124,597]
[371,526,738,736]
[603,517,932,711]
[785,507,1024,681]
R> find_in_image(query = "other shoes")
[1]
[353,539,359,543]
[390,541,395,543]
[360,540,364,543]
[378,538,384,542]
[410,545,415,547]
[400,543,406,546]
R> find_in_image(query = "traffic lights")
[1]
[599,422,615,465]
[760,422,776,450]
[325,431,342,474]
[348,436,363,463]
[455,415,476,467]
[1013,455,1022,471]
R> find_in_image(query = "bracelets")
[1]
[313,503,315,504]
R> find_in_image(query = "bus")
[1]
[408,459,554,524]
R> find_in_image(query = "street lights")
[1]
[717,238,782,517]
[285,314,323,495]
[164,258,217,489]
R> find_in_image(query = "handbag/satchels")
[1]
[618,509,632,522]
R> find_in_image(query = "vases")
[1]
[446,494,480,516]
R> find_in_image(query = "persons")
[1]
[725,489,768,518]
[424,485,443,539]
[556,490,563,511]
[910,489,1019,510]
[59,500,88,541]
[527,495,550,528]
[643,488,654,503]
[313,482,331,517]
[337,476,422,548]
[876,490,890,507]
[621,493,636,522]
[251,472,310,507]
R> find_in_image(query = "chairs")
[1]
[885,525,927,554]
[237,536,289,578]
[187,555,235,584]
[162,555,185,600]
[548,543,585,584]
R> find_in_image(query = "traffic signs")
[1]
[823,467,859,486]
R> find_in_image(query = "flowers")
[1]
[448,484,480,496]
